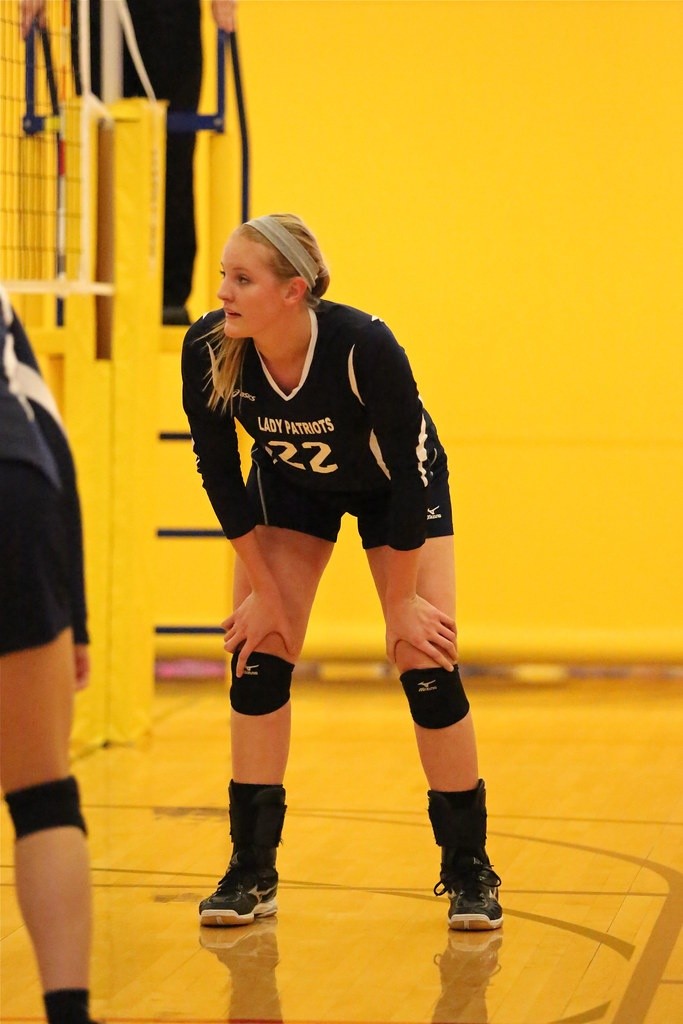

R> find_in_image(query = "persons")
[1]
[19,0,236,326]
[180,212,504,930]
[0,284,94,1024]
[197,917,504,1024]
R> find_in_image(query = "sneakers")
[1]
[433,856,503,931]
[199,926,280,974]
[198,851,278,925]
[434,931,503,995]
